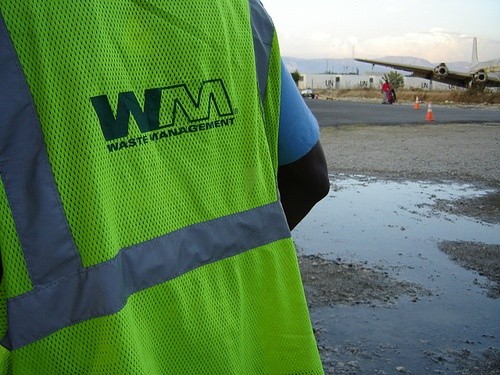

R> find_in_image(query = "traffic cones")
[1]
[413,96,420,110]
[425,101,435,121]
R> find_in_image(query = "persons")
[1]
[381,80,394,103]
[0,0,330,375]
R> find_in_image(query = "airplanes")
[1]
[354,36,500,94]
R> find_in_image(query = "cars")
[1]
[300,88,315,99]
